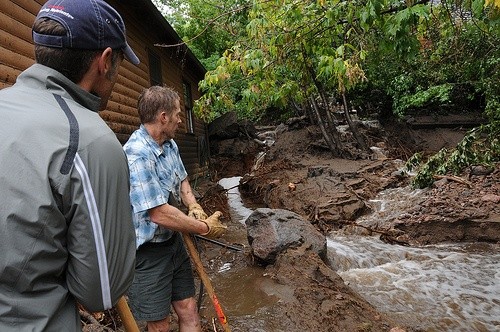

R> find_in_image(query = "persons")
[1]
[0,0,139,332]
[122,86,229,331]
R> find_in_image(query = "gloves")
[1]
[187,204,207,221]
[198,210,227,240]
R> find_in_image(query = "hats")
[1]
[31,1,139,66]
